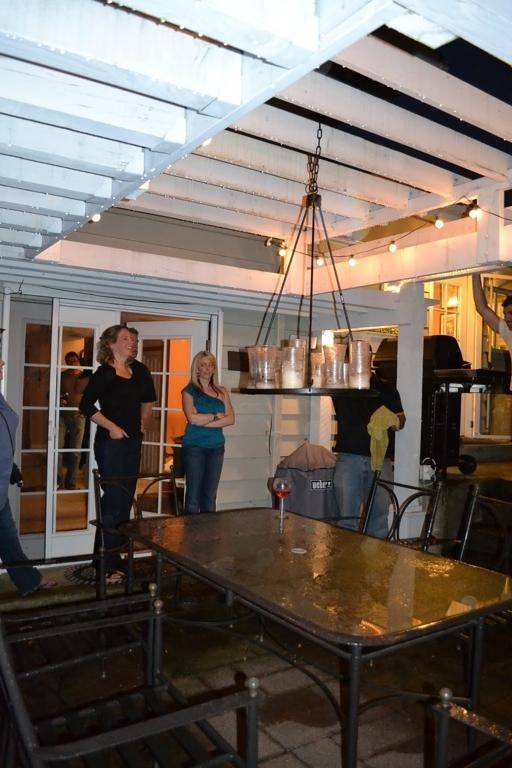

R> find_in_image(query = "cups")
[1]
[245,335,370,389]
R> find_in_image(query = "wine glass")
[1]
[272,477,296,520]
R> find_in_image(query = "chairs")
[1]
[357,470,444,558]
[90,465,182,679]
[458,482,511,573]
[1,583,256,768]
[431,687,512,768]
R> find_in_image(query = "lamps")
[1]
[265,194,512,268]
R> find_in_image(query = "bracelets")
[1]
[213,412,219,421]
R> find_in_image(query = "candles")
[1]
[247,336,371,390]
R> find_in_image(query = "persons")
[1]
[46,351,88,490]
[75,327,157,434]
[79,325,142,579]
[0,351,58,599]
[472,273,512,392]
[182,351,235,515]
[333,342,405,540]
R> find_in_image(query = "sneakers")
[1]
[92,568,127,584]
